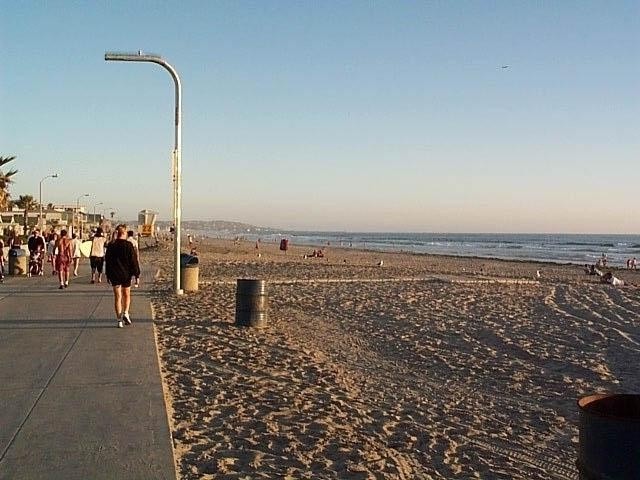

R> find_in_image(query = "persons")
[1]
[0,225,193,289]
[105,224,142,328]
[584,254,637,286]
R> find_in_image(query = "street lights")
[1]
[93,202,121,225]
[39,174,58,236]
[77,194,89,213]
[104,51,182,296]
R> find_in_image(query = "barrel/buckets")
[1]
[181,264,199,293]
[181,253,199,268]
[281,240,288,250]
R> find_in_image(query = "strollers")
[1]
[28,249,44,278]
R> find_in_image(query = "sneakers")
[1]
[118,313,131,328]
[90,280,94,283]
[59,285,64,289]
[65,282,68,288]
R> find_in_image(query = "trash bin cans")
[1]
[9,248,27,276]
[180,253,200,292]
